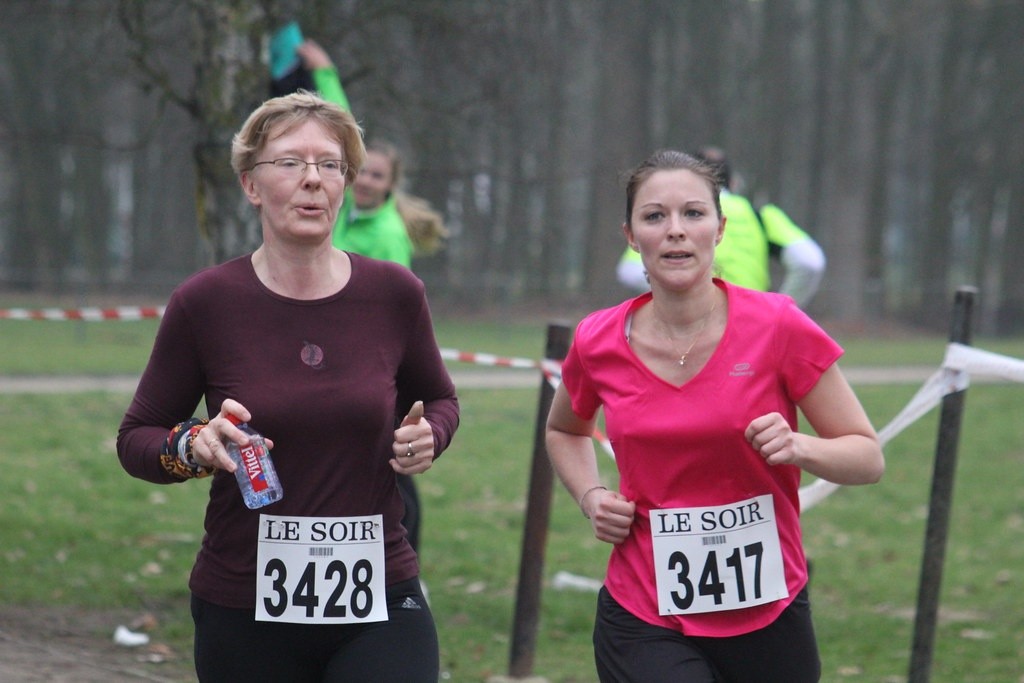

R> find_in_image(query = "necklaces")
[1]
[652,293,718,366]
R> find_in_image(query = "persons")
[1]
[545,146,885,683]
[294,39,451,269]
[614,147,827,306]
[115,89,462,683]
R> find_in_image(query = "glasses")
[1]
[250,158,350,180]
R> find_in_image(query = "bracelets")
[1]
[160,417,216,482]
[581,486,608,520]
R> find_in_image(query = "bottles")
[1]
[222,412,283,509]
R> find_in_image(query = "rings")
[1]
[406,442,415,457]
